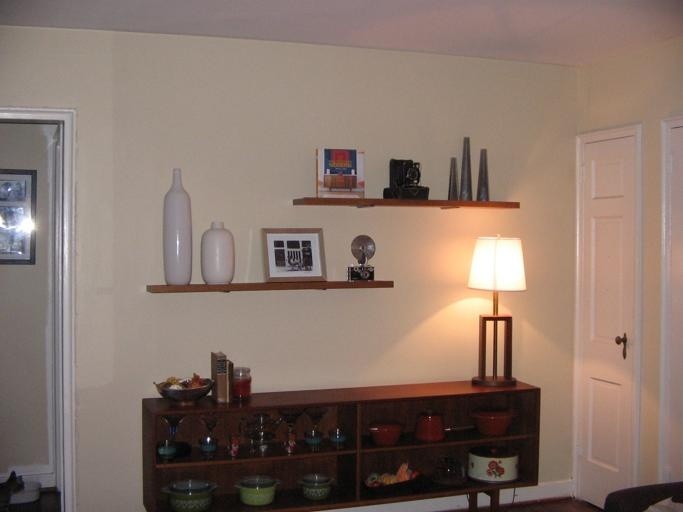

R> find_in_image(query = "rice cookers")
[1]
[466,446,519,483]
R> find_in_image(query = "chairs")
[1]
[602,475,681,512]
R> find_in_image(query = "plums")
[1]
[164,383,172,389]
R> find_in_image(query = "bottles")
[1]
[448,137,489,201]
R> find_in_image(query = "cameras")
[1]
[348,264,374,282]
[402,163,419,186]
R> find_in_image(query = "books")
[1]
[211,350,227,404]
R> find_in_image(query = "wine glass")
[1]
[159,406,346,459]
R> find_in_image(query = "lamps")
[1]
[465,234,525,388]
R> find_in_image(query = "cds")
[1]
[350,235,375,260]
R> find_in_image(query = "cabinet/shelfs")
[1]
[142,191,523,294]
[139,375,542,511]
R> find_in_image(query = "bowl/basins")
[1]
[10,482,42,505]
[297,474,335,501]
[156,379,214,406]
[232,475,281,505]
[367,406,519,447]
[160,479,218,512]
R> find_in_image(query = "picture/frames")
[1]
[259,225,325,283]
[0,170,38,268]
